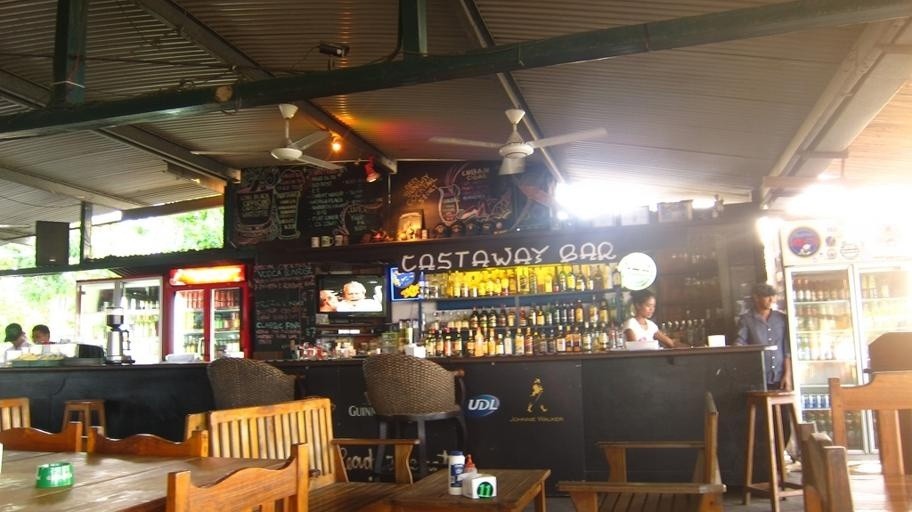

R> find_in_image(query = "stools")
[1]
[57,398,106,448]
[0,394,29,431]
[743,384,808,510]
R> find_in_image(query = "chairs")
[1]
[207,357,295,407]
[0,418,82,451]
[364,353,468,480]
[796,367,911,512]
[165,439,308,512]
[89,423,209,460]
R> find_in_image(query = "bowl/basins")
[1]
[166,354,194,362]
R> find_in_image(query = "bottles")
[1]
[406,223,415,240]
[447,449,477,496]
[398,318,420,346]
[669,247,720,320]
[661,317,706,343]
[801,411,861,448]
[793,332,856,362]
[129,313,157,336]
[804,391,831,409]
[421,262,626,358]
[104,291,159,310]
[791,273,905,332]
[183,312,240,360]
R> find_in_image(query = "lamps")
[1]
[363,160,382,183]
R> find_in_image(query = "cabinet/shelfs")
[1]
[390,235,727,351]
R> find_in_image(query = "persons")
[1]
[32,324,55,345]
[4,322,32,360]
[623,291,677,348]
[318,290,339,312]
[339,281,383,313]
[734,282,802,472]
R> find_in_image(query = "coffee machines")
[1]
[104,313,135,365]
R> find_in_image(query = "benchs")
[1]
[185,398,419,510]
[557,391,727,511]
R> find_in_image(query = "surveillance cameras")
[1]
[320,39,350,57]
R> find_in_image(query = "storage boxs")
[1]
[658,199,693,224]
[619,204,652,227]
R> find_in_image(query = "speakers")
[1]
[36,219,70,266]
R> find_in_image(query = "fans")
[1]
[422,108,609,178]
[193,103,345,177]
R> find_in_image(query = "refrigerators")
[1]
[78,276,162,363]
[162,259,252,363]
[773,213,911,456]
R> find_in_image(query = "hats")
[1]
[4,324,22,343]
[752,284,777,296]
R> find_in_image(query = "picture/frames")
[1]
[394,207,424,243]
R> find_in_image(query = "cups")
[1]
[298,348,321,359]
[334,235,343,246]
[321,235,334,247]
[708,335,726,347]
[311,236,320,247]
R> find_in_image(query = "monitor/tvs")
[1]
[315,273,388,318]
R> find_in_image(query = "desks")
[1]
[390,466,549,511]
[1,447,288,511]
